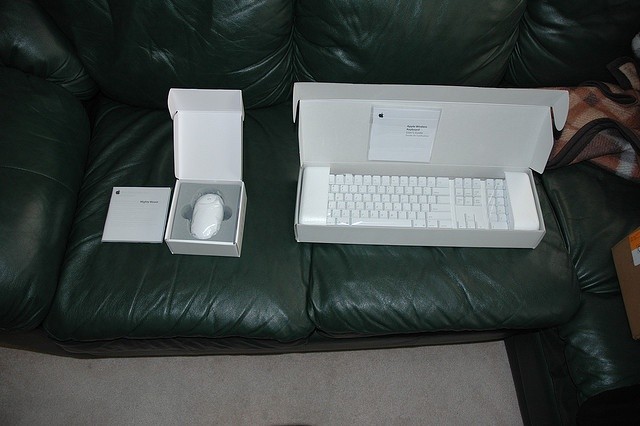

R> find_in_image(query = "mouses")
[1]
[191,194,224,240]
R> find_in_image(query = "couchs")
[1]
[0,0,640,347]
[0,0,640,426]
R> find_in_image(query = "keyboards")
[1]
[325,174,511,230]
